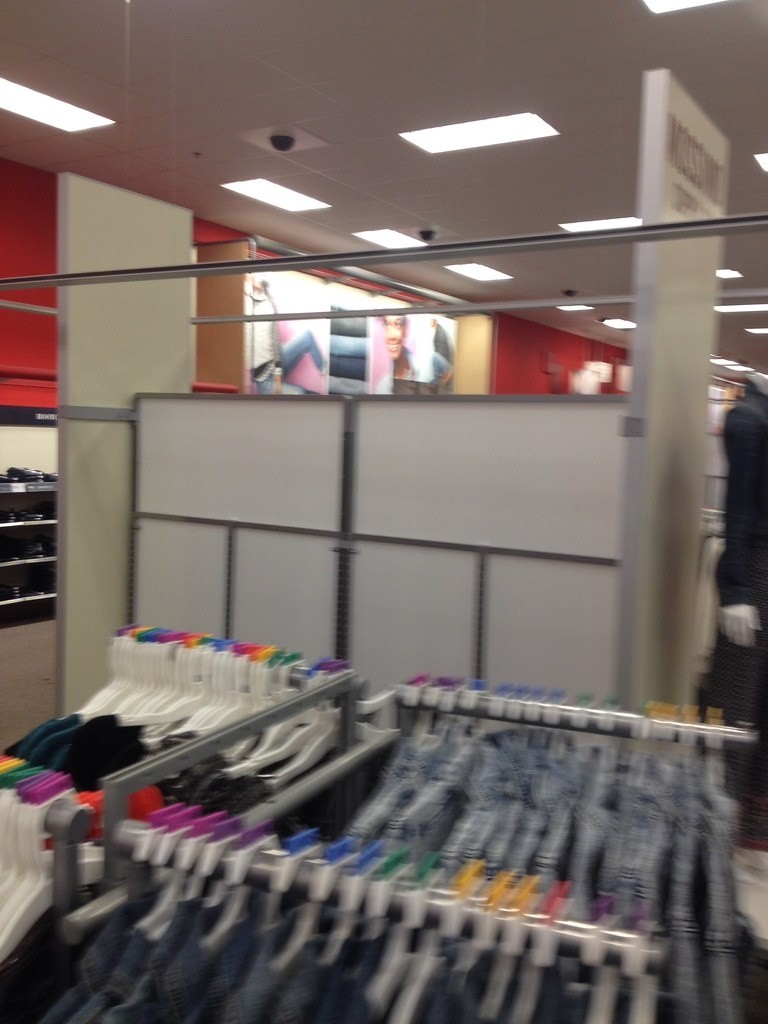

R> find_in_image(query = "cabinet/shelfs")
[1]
[0,404,61,760]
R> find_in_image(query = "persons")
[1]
[383,316,417,380]
[696,372,768,1024]
[429,317,455,387]
[244,255,285,395]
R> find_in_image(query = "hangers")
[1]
[0,625,748,1024]
